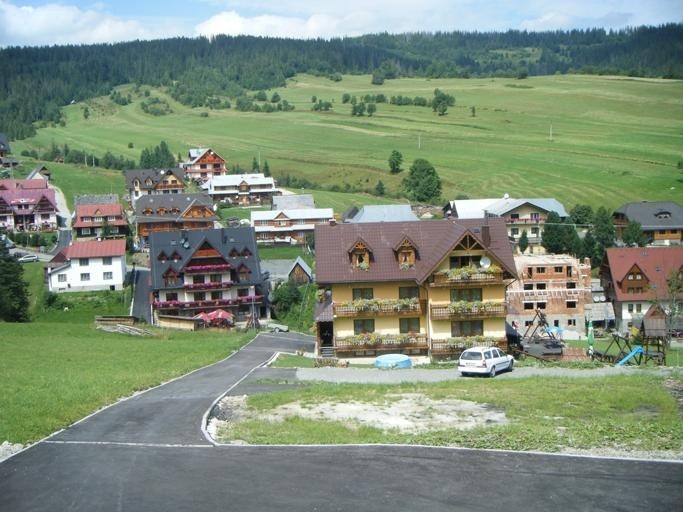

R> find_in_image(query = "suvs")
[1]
[457,346,517,380]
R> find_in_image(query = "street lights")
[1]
[249,281,257,334]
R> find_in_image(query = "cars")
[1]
[4,241,39,264]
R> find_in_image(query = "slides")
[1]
[615,347,644,367]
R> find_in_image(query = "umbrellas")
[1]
[587,319,593,359]
